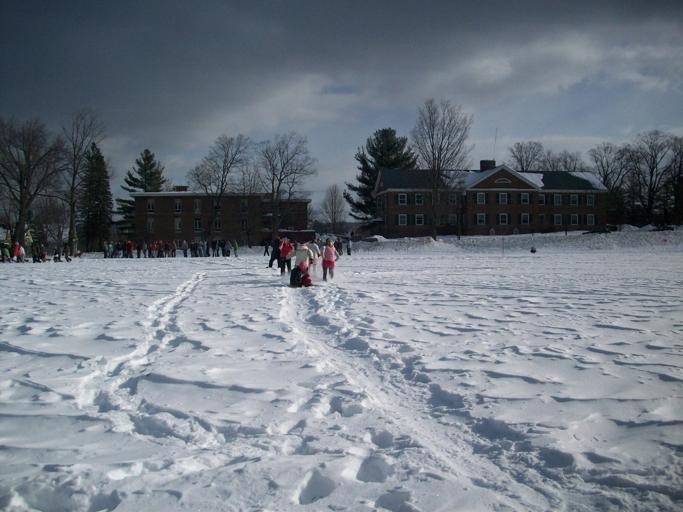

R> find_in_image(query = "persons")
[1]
[76,238,239,259]
[248,236,352,286]
[1,237,72,263]
